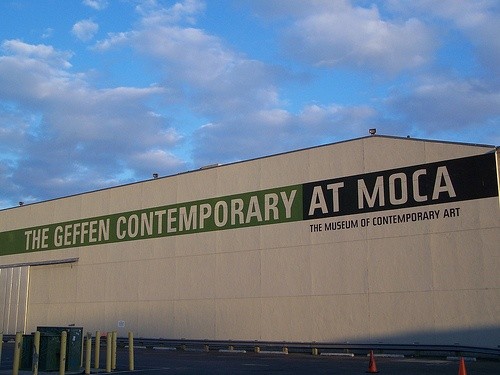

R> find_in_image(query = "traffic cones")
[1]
[365,349,377,373]
[455,358,468,375]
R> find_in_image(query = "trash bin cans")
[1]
[36,325,83,372]
[20,332,68,372]
